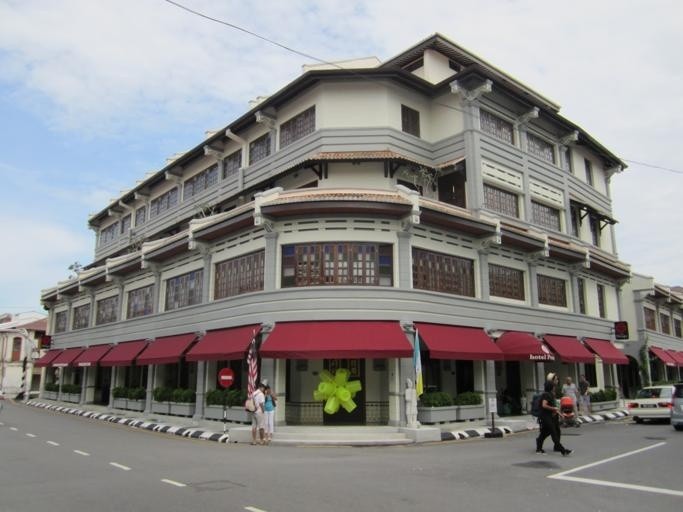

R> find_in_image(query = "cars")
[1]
[670,381,682,431]
[628,384,675,424]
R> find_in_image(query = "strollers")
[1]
[559,397,580,428]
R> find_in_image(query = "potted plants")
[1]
[589,387,617,411]
[418,393,457,422]
[44,382,252,424]
[458,392,485,421]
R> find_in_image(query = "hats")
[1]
[547,372,556,382]
[260,379,268,387]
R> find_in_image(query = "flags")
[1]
[411,330,423,398]
[246,333,257,400]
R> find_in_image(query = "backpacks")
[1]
[531,394,543,416]
[245,398,256,411]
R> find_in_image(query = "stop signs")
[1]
[218,367,235,389]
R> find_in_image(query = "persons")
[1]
[404,378,418,425]
[576,375,591,416]
[560,376,579,417]
[535,381,575,456]
[554,379,563,409]
[261,385,277,442]
[249,383,266,446]
[544,370,562,451]
